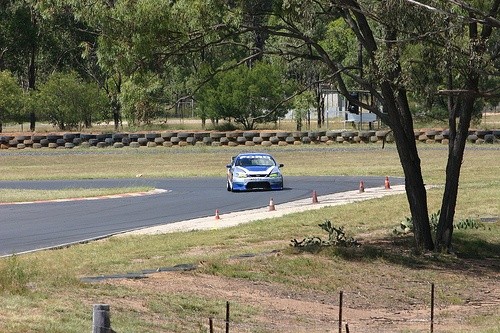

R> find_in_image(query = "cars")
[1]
[226,151,285,193]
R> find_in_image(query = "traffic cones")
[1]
[214,208,221,220]
[384,176,392,189]
[268,197,276,211]
[358,179,365,193]
[312,188,319,203]
